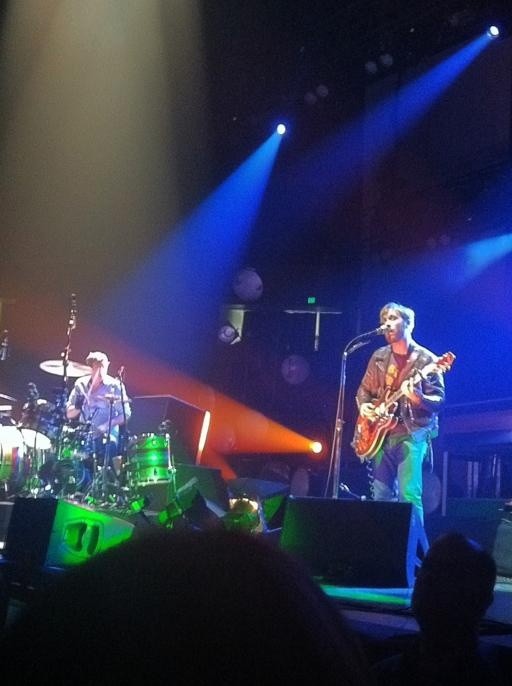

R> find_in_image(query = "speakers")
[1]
[492,517,512,577]
[4,497,134,568]
[280,495,430,587]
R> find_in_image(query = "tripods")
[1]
[18,392,130,507]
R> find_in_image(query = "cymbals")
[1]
[39,359,92,376]
[1,394,17,403]
[95,394,132,402]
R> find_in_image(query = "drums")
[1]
[0,413,29,500]
[52,459,88,494]
[60,422,92,461]
[17,398,59,449]
[127,433,173,485]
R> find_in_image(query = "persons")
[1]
[66,353,131,497]
[355,304,446,564]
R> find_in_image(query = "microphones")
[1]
[118,365,125,377]
[359,325,391,340]
[71,293,77,314]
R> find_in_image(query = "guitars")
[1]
[354,351,456,459]
